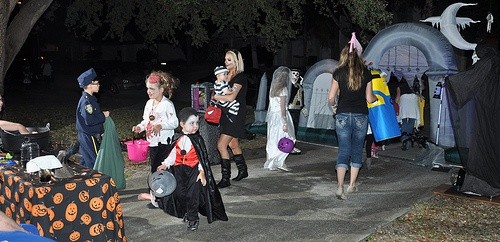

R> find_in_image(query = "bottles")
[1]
[432,81,442,100]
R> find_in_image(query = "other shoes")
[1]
[183,214,199,231]
[346,186,358,193]
[336,185,347,199]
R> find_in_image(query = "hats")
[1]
[77,68,97,88]
[214,66,230,76]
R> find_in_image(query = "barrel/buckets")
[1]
[20,142,39,169]
[148,170,177,198]
[124,132,150,161]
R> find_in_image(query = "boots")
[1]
[232,152,248,181]
[216,157,231,188]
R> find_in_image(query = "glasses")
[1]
[91,81,100,85]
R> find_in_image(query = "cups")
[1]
[39,169,52,184]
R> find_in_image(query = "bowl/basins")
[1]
[0,123,51,153]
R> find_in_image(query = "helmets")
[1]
[278,137,295,153]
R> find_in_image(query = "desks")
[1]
[0,150,128,242]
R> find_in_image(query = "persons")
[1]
[211,49,256,188]
[287,68,308,154]
[154,107,228,231]
[329,32,378,200]
[77,68,109,168]
[214,65,240,123]
[0,210,56,242]
[362,60,429,168]
[0,97,29,134]
[131,72,179,208]
[264,67,297,171]
